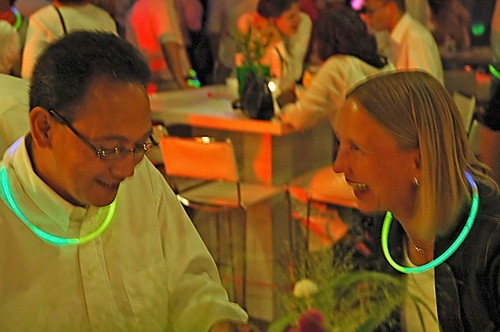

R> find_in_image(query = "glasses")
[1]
[48,108,160,161]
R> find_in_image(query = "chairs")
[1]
[305,90,476,276]
[158,136,296,309]
[283,169,315,281]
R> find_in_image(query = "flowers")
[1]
[224,11,274,66]
[292,278,330,332]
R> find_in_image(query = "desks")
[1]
[149,84,334,322]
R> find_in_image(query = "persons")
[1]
[0,30,263,332]
[0,0,500,226]
[290,70,500,332]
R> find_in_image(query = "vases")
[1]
[235,65,271,98]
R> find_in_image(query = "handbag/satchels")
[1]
[239,70,275,121]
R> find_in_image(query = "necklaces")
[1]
[409,237,435,254]
